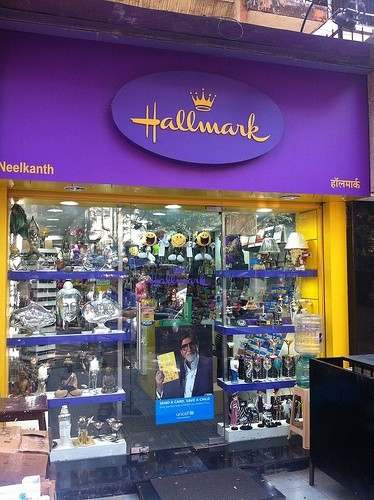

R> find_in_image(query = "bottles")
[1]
[296,356,315,388]
[293,314,322,356]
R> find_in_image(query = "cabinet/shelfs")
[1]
[9,269,127,462]
[214,269,317,443]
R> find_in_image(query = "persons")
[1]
[277,293,283,305]
[246,296,257,308]
[229,392,301,425]
[10,370,31,397]
[55,281,81,323]
[155,333,213,399]
[54,353,82,398]
[102,366,118,393]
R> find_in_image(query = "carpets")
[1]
[149,468,273,500]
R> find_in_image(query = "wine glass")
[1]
[284,354,293,380]
[253,358,262,382]
[263,356,272,382]
[283,337,294,355]
[273,355,283,381]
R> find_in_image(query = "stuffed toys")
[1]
[192,232,215,260]
[163,233,192,262]
[138,232,159,261]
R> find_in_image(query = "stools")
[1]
[0,394,50,466]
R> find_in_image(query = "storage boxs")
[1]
[0,427,57,500]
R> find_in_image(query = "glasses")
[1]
[180,341,197,350]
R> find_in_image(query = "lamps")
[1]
[284,232,309,265]
[310,1,365,38]
[258,238,280,261]
